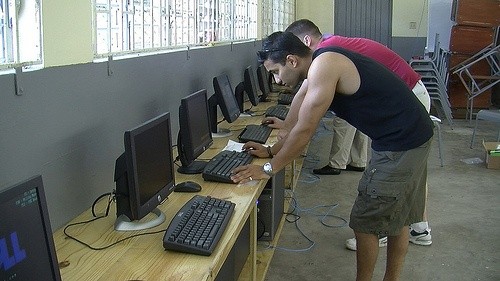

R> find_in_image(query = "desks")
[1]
[52,81,310,281]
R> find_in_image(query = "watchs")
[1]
[263,162,274,177]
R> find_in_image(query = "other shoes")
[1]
[313,165,341,175]
[342,164,365,172]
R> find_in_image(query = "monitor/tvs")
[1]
[0,64,274,281]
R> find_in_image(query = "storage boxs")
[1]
[485,150,500,169]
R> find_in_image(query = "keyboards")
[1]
[265,94,294,120]
[238,125,273,144]
[163,194,235,256]
[202,150,254,185]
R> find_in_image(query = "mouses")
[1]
[172,181,202,192]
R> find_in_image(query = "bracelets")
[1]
[266,146,273,159]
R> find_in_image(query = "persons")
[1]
[285,19,432,251]
[313,114,367,175]
[230,31,434,281]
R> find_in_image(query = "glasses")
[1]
[256,48,294,60]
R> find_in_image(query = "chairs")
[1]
[471,109,500,150]
[409,33,454,130]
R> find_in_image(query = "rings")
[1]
[249,176,252,181]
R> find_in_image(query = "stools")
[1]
[429,115,443,167]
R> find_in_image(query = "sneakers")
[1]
[345,235,388,251]
[408,224,432,246]
